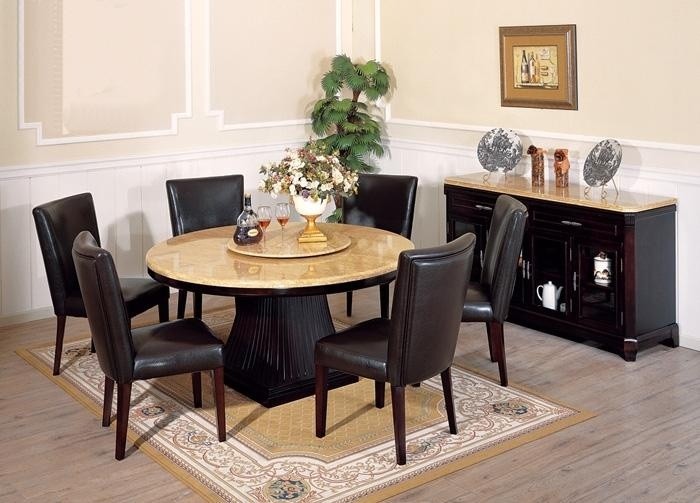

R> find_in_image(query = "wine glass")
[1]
[275,204,291,245]
[545,62,557,85]
[256,205,272,245]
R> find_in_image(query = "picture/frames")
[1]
[499,24,578,110]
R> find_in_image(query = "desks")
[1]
[146,223,419,408]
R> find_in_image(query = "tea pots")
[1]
[537,280,563,310]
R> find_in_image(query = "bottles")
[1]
[520,50,540,84]
[233,192,265,246]
[555,148,570,187]
[531,149,544,188]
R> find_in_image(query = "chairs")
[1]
[460,195,528,385]
[14,303,601,503]
[315,233,476,465]
[344,175,417,320]
[72,231,226,461]
[33,193,169,375]
[166,175,244,318]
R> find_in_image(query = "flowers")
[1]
[255,136,361,204]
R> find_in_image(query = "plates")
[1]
[476,128,524,173]
[583,139,622,191]
[515,83,540,87]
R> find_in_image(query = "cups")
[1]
[593,251,613,286]
[559,302,566,313]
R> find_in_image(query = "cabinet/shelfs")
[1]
[444,171,679,362]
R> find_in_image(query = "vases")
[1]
[291,194,329,243]
